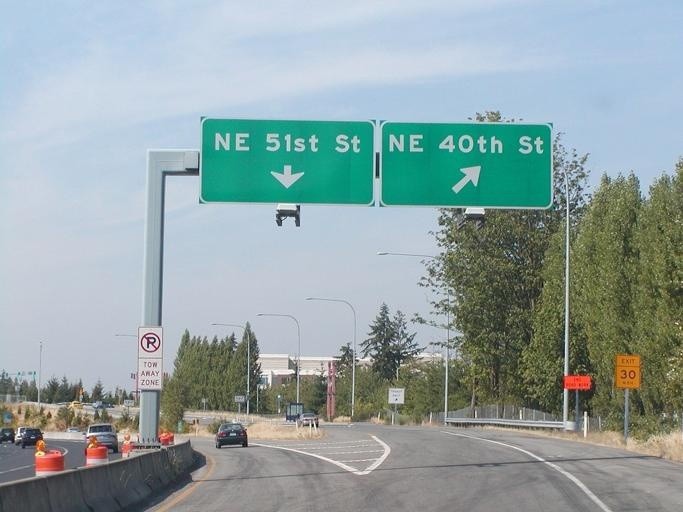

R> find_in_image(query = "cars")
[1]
[72,401,114,409]
[83,423,119,454]
[15,427,26,444]
[65,427,81,432]
[214,423,248,448]
[22,427,43,448]
[298,411,319,427]
[0,428,15,444]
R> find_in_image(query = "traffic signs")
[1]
[380,120,553,209]
[199,116,376,207]
[616,355,640,389]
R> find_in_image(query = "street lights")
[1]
[377,252,448,426]
[116,334,137,337]
[257,313,300,404]
[305,297,356,415]
[211,323,249,415]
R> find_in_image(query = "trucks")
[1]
[286,403,304,422]
